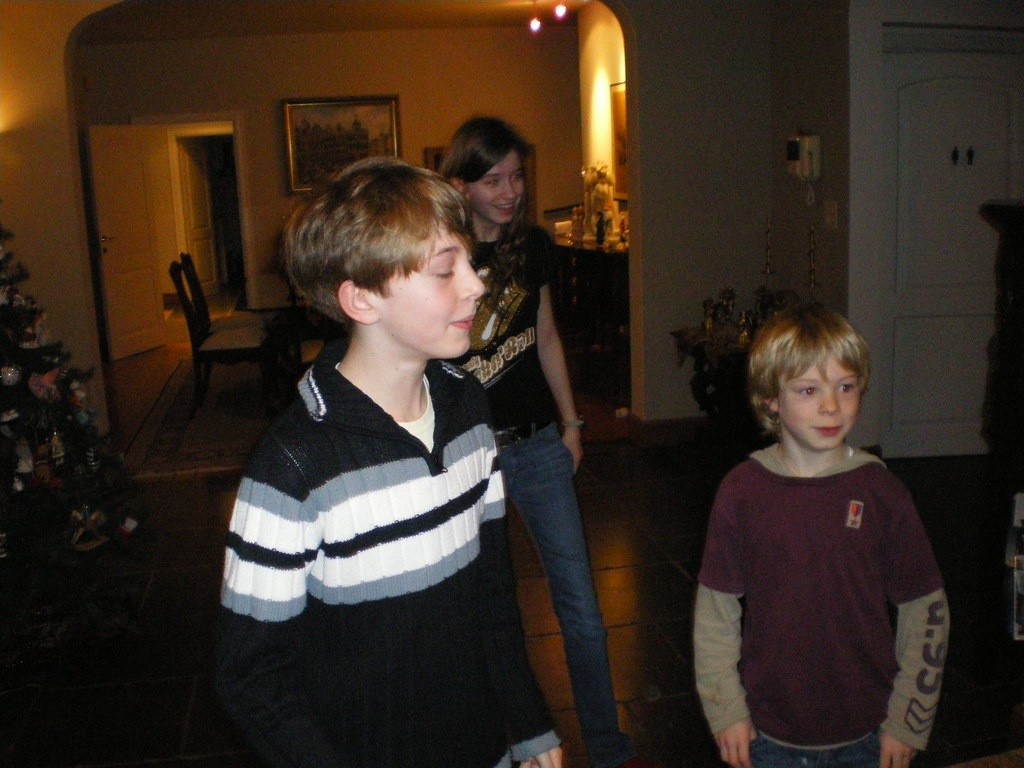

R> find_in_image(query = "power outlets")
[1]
[823,199,838,233]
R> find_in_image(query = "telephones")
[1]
[785,135,822,181]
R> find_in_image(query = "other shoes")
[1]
[620,756,662,768]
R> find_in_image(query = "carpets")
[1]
[123,358,317,483]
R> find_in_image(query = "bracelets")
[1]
[561,420,585,428]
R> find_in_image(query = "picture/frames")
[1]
[425,144,538,224]
[280,94,402,196]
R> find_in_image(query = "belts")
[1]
[493,413,558,448]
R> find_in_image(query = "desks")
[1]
[555,208,629,386]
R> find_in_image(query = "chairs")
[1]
[168,260,286,431]
[179,252,282,409]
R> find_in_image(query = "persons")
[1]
[691,297,951,768]
[206,154,563,768]
[428,117,664,768]
